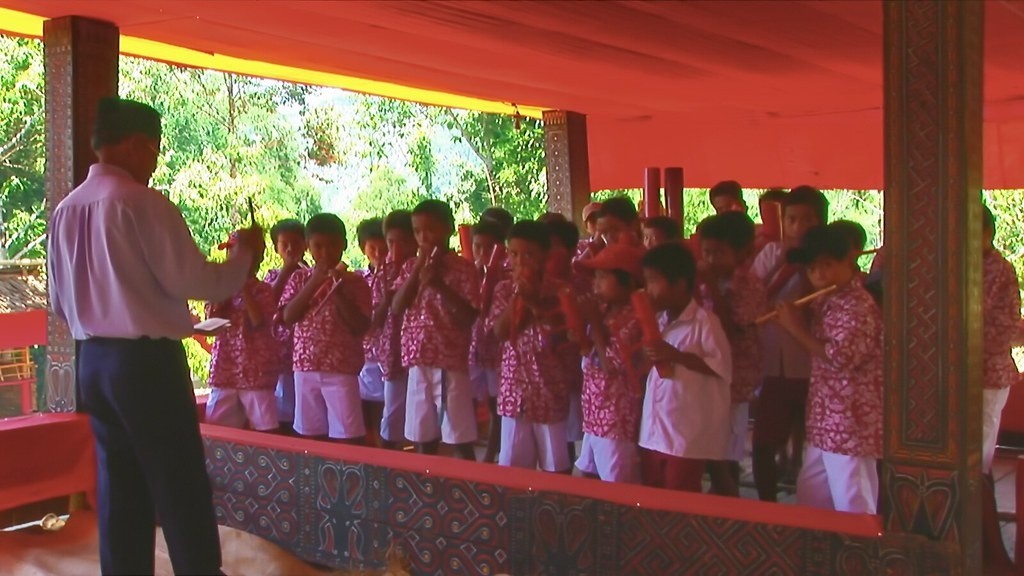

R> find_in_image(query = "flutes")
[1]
[753,283,840,325]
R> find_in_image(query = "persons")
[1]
[187,177,1024,513]
[47,101,253,575]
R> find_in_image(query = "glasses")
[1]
[146,142,165,167]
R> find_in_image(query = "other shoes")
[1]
[741,470,759,487]
[775,461,800,490]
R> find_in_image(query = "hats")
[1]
[217,229,267,254]
[582,202,603,221]
[785,228,850,262]
